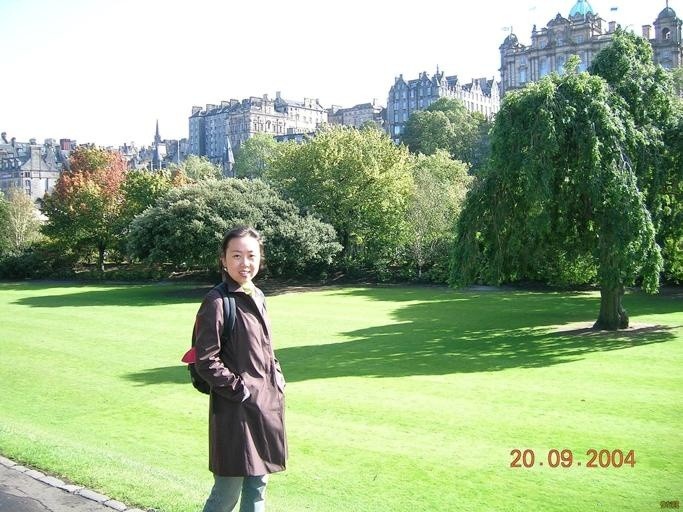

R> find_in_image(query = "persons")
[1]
[180,224,290,512]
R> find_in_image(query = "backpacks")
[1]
[181,282,237,394]
